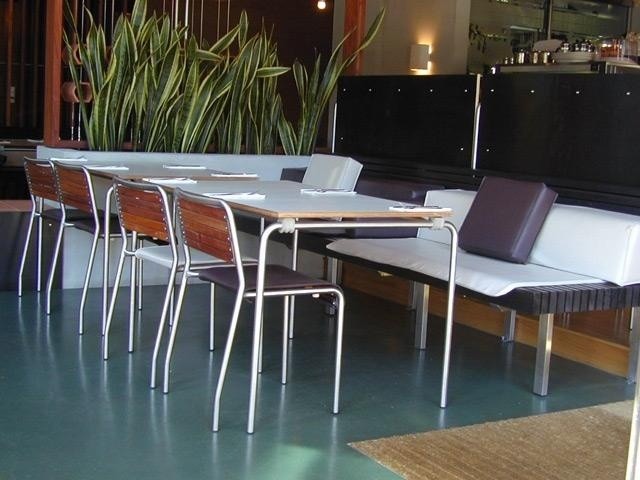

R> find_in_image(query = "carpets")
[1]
[347,400,634,478]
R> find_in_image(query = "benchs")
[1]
[226,169,443,317]
[232,168,640,398]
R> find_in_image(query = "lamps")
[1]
[409,44,431,69]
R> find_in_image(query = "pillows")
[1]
[302,154,363,221]
[458,175,558,263]
